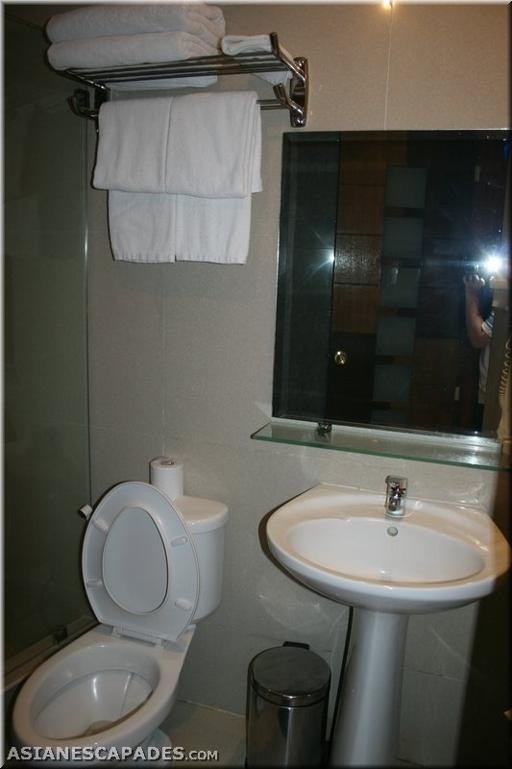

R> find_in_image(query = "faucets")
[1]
[385,476,407,515]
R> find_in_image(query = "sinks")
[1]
[266,482,511,615]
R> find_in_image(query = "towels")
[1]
[45,4,293,266]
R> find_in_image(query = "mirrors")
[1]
[272,128,512,440]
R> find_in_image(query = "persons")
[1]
[461,265,496,407]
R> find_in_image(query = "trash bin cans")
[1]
[245,640,332,769]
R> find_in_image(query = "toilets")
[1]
[12,480,229,768]
